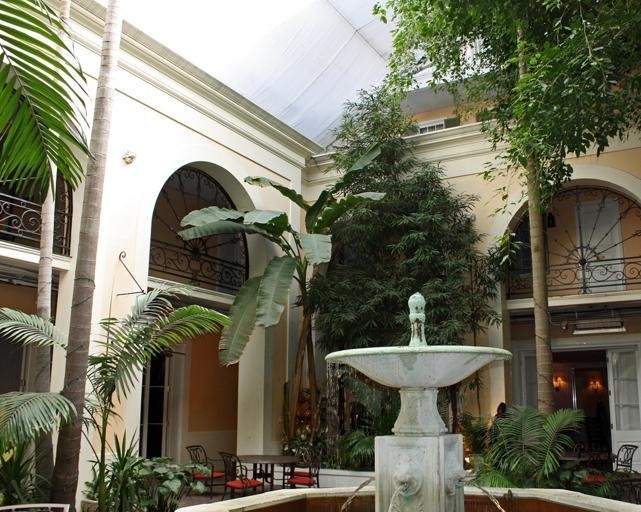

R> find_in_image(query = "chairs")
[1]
[572,442,639,504]
[184,444,225,500]
[287,444,323,488]
[218,450,265,501]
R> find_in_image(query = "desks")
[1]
[230,455,300,499]
[558,451,618,472]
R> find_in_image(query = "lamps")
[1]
[560,319,568,330]
[573,326,626,335]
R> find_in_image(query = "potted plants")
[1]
[80,466,111,512]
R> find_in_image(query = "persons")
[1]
[490,402,510,439]
